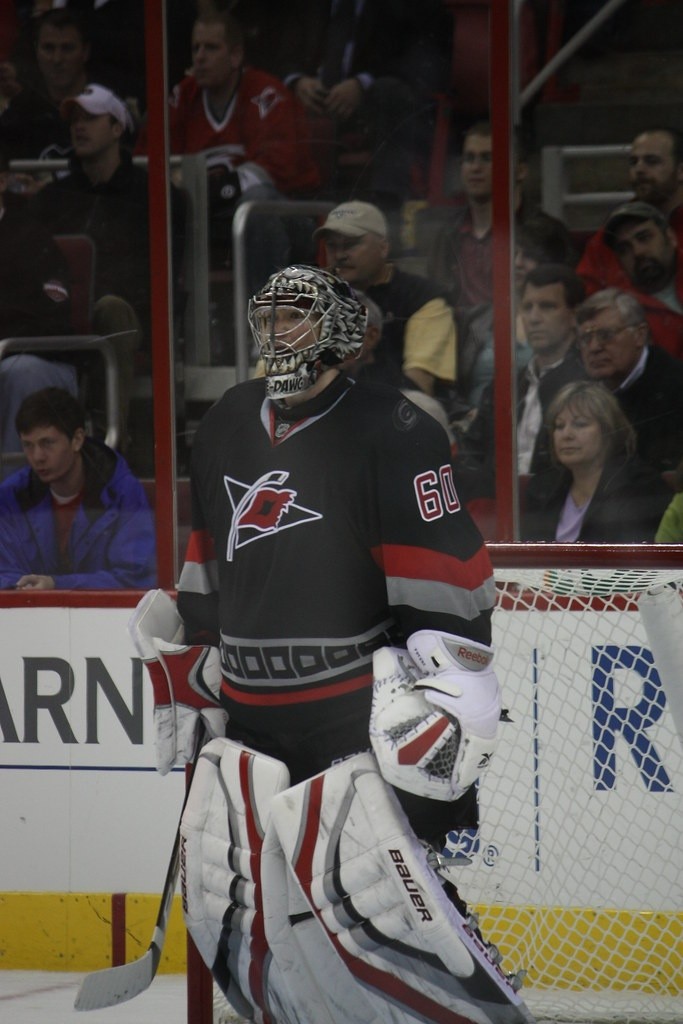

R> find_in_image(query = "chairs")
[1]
[51,235,96,331]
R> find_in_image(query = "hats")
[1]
[311,200,386,242]
[58,83,127,132]
[602,201,667,256]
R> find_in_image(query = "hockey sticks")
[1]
[71,713,205,1013]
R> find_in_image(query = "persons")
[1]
[1,2,682,589]
[121,261,521,1024]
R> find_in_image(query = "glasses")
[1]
[572,321,644,350]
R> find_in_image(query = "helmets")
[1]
[246,264,368,401]
